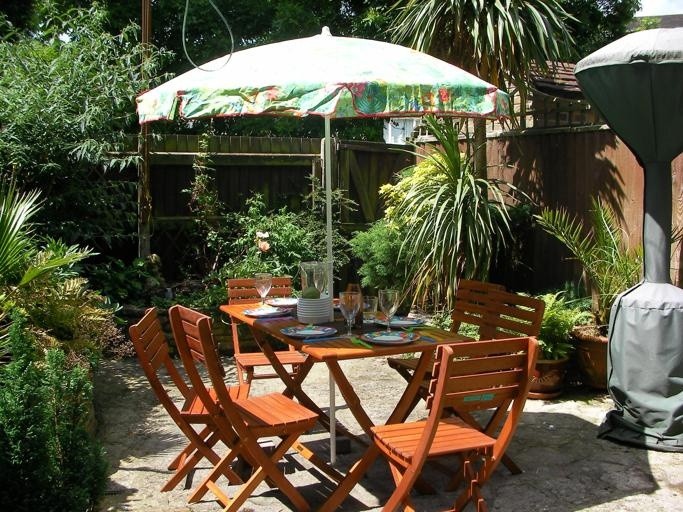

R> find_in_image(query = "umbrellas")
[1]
[137,26,513,327]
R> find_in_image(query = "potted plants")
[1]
[504,195,683,399]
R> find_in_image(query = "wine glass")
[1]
[337,290,361,339]
[376,289,400,337]
[361,295,377,333]
[253,273,273,308]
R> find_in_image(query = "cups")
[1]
[299,260,326,299]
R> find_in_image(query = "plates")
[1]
[376,317,423,328]
[279,323,338,338]
[241,307,293,318]
[359,329,420,346]
[296,295,331,325]
[264,297,297,307]
